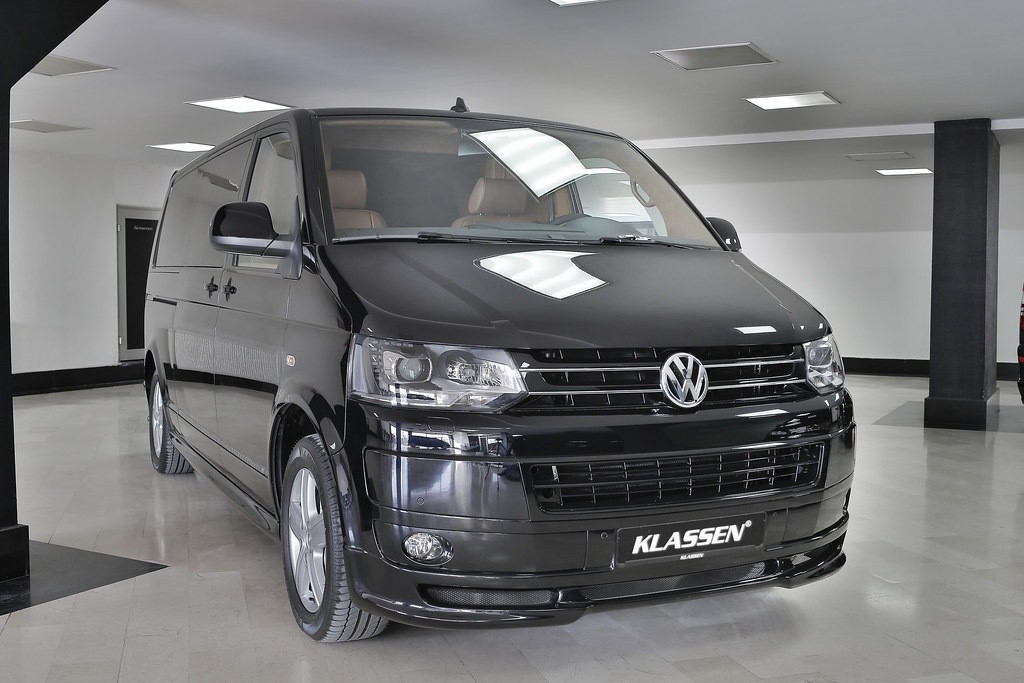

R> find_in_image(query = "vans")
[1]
[138,95,858,644]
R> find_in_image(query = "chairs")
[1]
[329,168,389,229]
[449,176,551,228]
